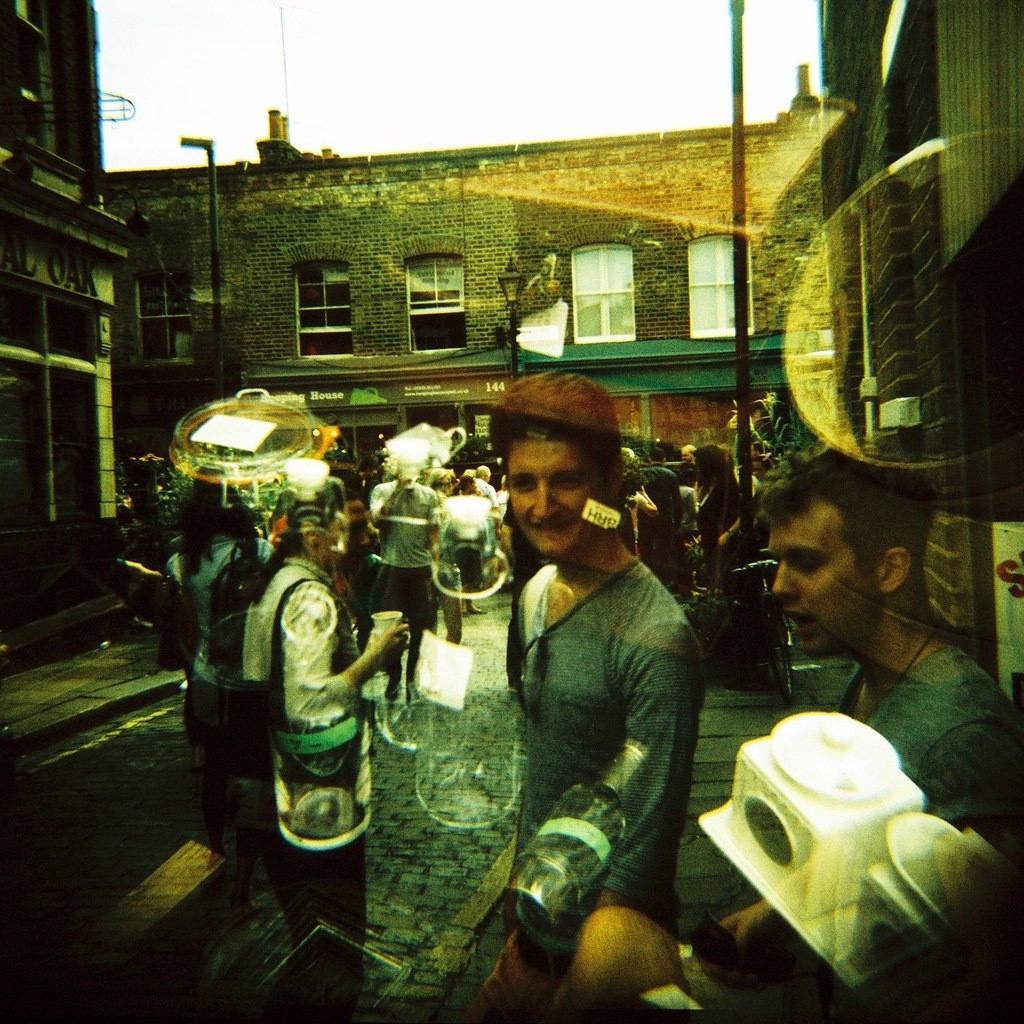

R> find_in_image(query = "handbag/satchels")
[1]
[272,699,375,849]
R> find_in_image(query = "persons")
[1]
[713,451,1024,1024]
[167,469,273,901]
[464,372,702,1020]
[232,492,406,1021]
[125,443,764,698]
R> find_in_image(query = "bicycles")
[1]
[730,549,794,705]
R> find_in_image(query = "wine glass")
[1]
[416,545,526,829]
[374,588,454,751]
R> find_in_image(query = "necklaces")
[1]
[848,630,938,717]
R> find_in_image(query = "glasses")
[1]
[690,910,796,984]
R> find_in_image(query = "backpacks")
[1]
[207,538,266,667]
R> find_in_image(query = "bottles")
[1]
[498,735,654,919]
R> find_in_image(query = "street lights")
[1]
[181,137,225,399]
[496,256,526,378]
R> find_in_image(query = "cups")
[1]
[372,610,403,637]
